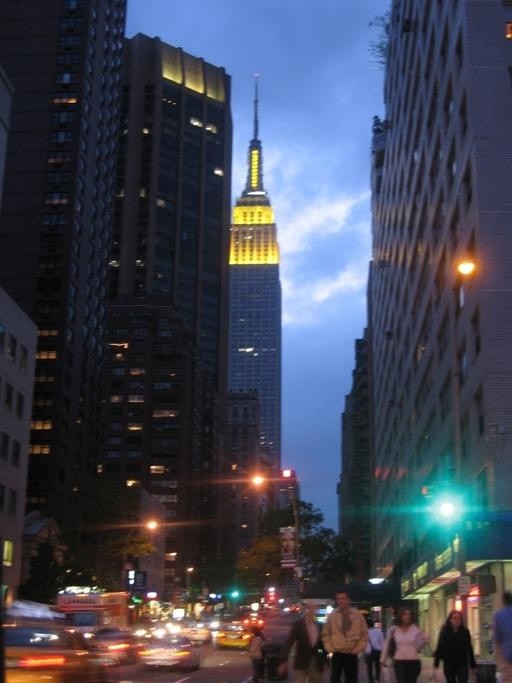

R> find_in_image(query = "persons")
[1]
[487,591,512,683]
[246,587,479,682]
[279,531,295,556]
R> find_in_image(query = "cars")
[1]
[176,616,255,649]
[138,633,199,671]
[86,625,136,665]
[2,622,120,683]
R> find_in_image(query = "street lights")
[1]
[120,520,156,592]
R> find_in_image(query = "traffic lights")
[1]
[420,481,460,525]
[231,590,239,598]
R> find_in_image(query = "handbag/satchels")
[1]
[388,626,396,656]
[369,648,381,659]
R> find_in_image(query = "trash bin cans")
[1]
[261,644,288,683]
[477,664,495,683]
